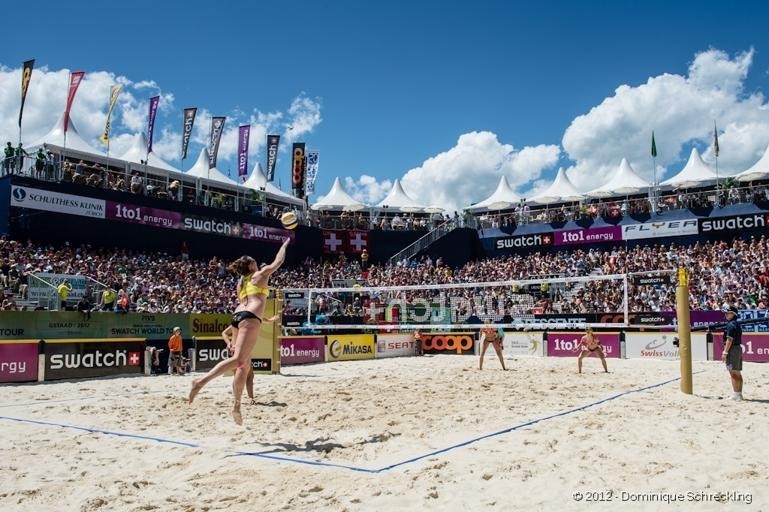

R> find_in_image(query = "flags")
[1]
[651,132,657,157]
[714,123,720,155]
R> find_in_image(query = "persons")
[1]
[179,357,190,373]
[188,237,291,424]
[721,306,743,402]
[145,346,164,375]
[572,327,607,373]
[222,315,280,399]
[0,142,769,335]
[478,319,508,369]
[168,326,183,375]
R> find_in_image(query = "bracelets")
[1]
[723,351,728,355]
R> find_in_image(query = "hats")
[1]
[721,305,739,316]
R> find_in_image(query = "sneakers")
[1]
[727,393,744,401]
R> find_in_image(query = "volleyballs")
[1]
[280,213,297,229]
